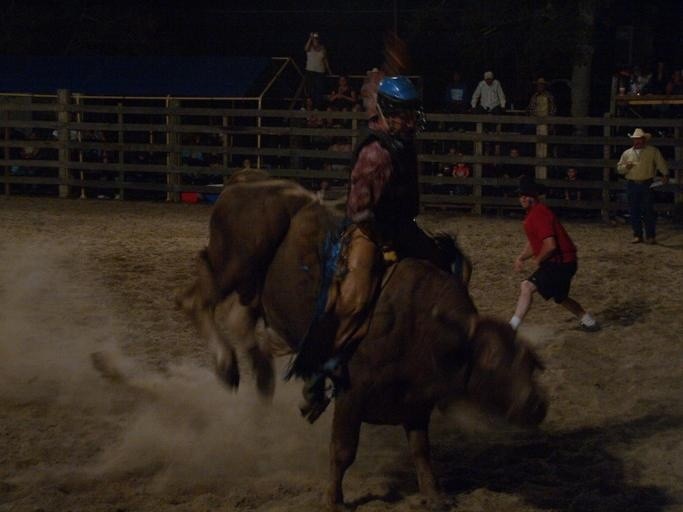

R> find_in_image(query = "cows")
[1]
[177,166,551,511]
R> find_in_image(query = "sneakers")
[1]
[632,237,640,244]
[575,322,600,332]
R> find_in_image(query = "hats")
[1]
[628,128,651,138]
[514,175,548,197]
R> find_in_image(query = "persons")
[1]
[78,130,108,199]
[299,39,469,409]
[243,159,251,169]
[616,128,668,244]
[21,132,37,177]
[508,175,602,332]
[302,32,681,217]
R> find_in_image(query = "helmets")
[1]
[377,75,418,102]
